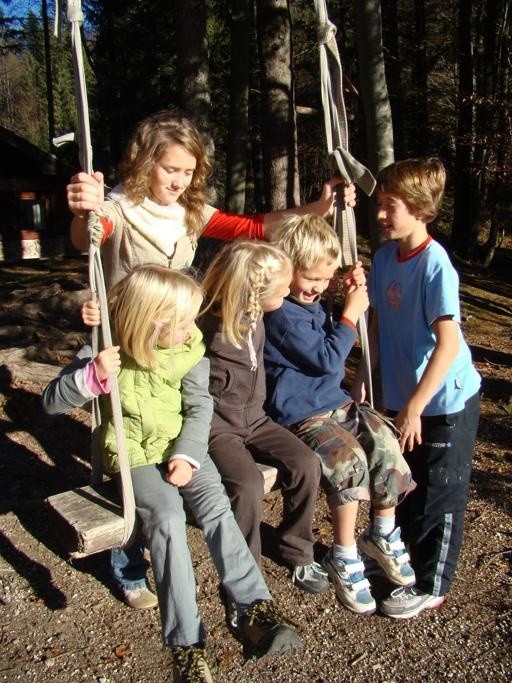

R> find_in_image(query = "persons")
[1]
[65,108,357,612]
[40,261,309,682]
[79,236,342,596]
[264,210,420,616]
[349,154,487,622]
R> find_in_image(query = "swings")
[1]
[45,1,380,561]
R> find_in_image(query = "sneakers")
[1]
[237,599,303,660]
[291,527,445,619]
[119,585,158,609]
[171,640,213,683]
